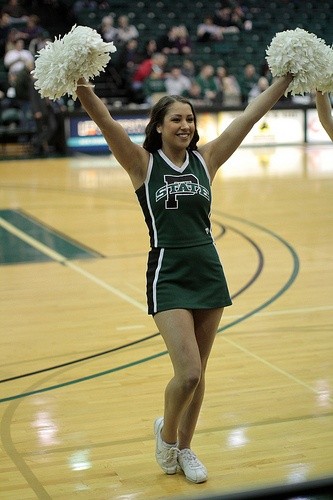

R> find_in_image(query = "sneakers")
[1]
[177,449,207,484]
[154,416,181,474]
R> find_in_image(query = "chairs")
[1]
[0,0,333,145]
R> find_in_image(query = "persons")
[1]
[315,88,333,143]
[72,0,318,111]
[0,0,71,158]
[60,73,299,484]
